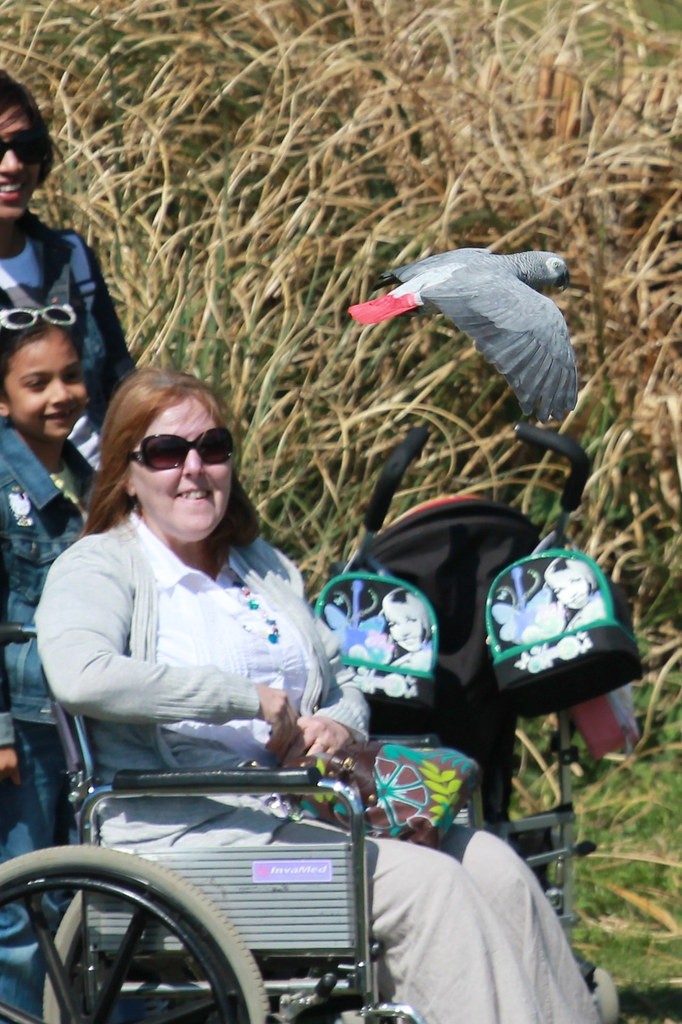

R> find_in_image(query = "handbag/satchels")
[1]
[484,531,642,719]
[315,530,439,738]
[271,722,482,851]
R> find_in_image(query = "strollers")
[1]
[307,422,589,902]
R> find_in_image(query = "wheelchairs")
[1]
[1,628,624,1024]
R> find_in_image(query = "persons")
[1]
[1,68,134,1024]
[34,362,600,1024]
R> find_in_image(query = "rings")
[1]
[314,741,328,749]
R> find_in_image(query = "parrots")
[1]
[347,247,579,424]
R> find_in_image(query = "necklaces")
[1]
[221,567,280,643]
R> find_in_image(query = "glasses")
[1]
[0,129,48,166]
[130,427,235,471]
[0,303,76,330]
